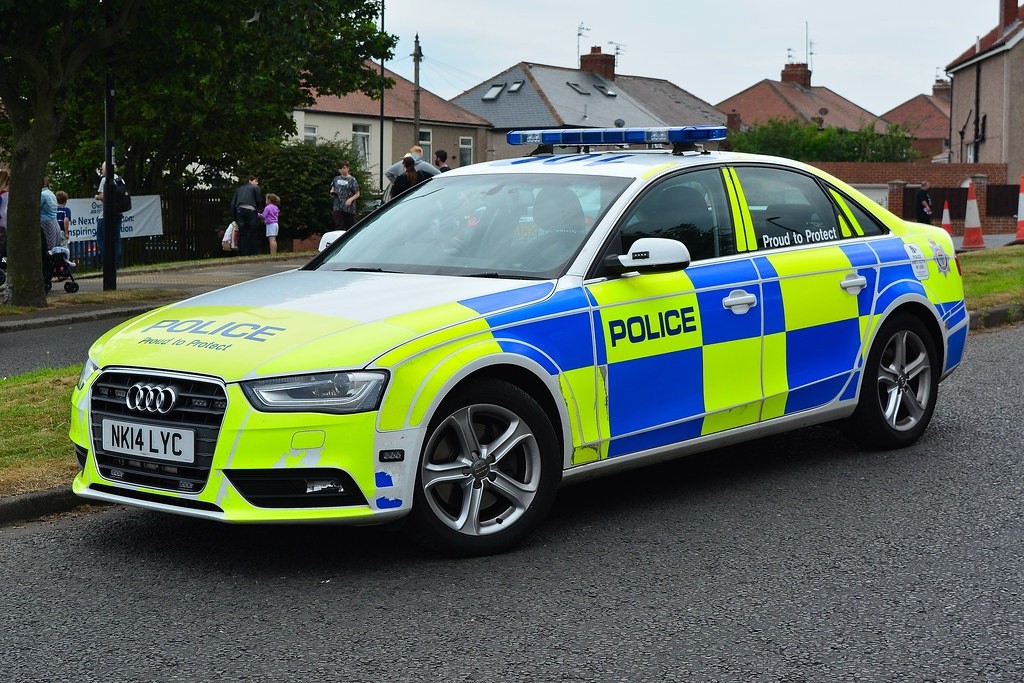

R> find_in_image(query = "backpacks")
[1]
[99,174,132,214]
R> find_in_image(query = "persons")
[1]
[392,157,434,198]
[39,174,62,251]
[329,161,361,233]
[384,146,442,182]
[256,193,281,255]
[915,181,933,224]
[433,149,451,175]
[0,169,9,270]
[94,161,126,270]
[54,190,72,279]
[230,175,263,255]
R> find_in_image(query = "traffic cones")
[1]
[959,178,986,249]
[941,200,953,237]
[1015,175,1024,240]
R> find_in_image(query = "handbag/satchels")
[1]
[220,221,240,253]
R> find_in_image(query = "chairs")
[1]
[644,186,715,263]
[518,186,588,274]
[760,203,817,249]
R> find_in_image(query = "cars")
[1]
[67,123,971,562]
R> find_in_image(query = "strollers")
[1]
[38,226,79,295]
